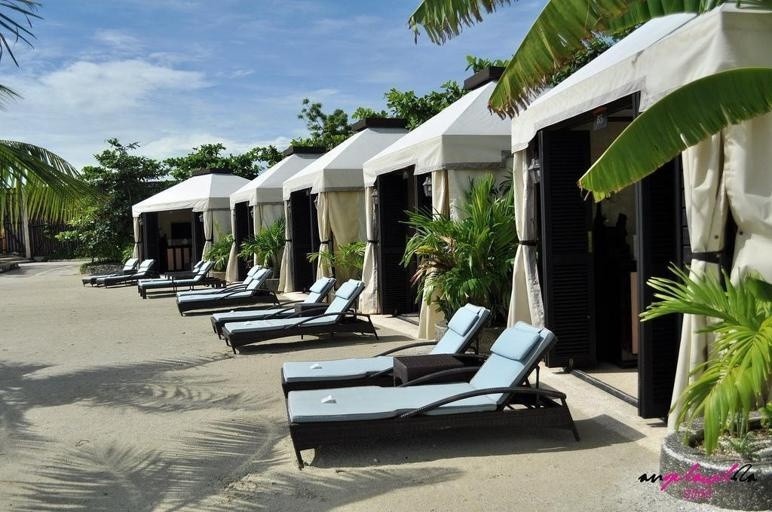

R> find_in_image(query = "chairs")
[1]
[138,259,380,354]
[281,304,491,402]
[81,257,157,288]
[285,321,580,473]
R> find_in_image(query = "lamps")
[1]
[528,158,540,185]
[370,189,378,205]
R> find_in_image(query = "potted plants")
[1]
[638,259,772,512]
[237,215,287,294]
[397,168,516,354]
[204,217,234,281]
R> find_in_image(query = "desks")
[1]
[391,355,488,387]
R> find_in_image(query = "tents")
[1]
[130,0,770,431]
